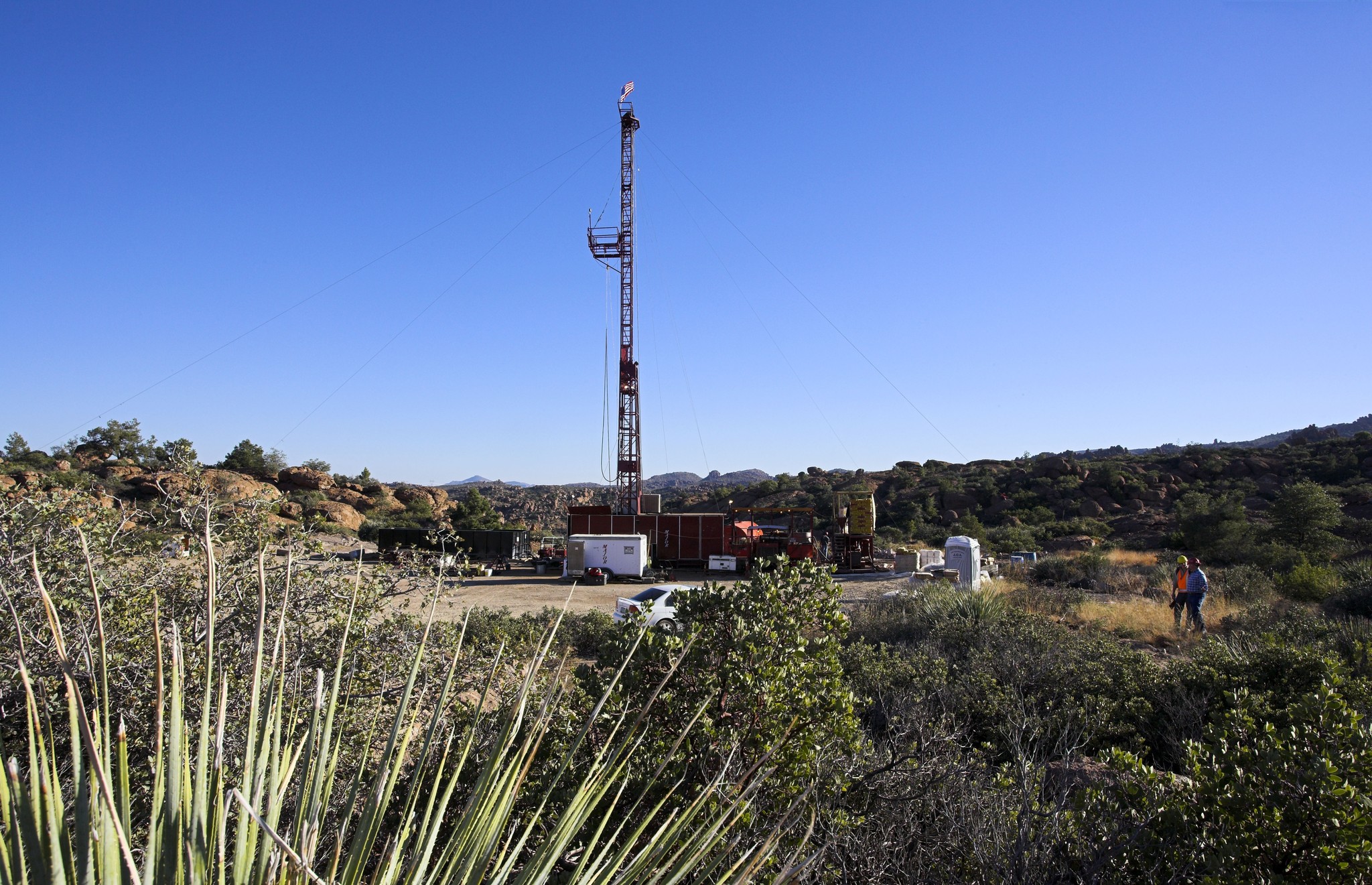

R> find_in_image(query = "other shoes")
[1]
[1201,630,1207,634]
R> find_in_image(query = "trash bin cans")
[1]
[1023,552,1037,561]
[1012,551,1017,556]
[1016,552,1025,558]
[1011,556,1024,570]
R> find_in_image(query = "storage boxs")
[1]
[709,555,737,571]
[485,569,492,577]
[586,574,607,585]
[349,549,365,560]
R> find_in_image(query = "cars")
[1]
[613,584,728,633]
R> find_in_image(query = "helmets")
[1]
[1186,558,1202,565]
[1177,556,1187,563]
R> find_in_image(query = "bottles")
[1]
[647,556,652,568]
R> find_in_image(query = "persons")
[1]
[1170,555,1193,633]
[819,532,829,561]
[1187,557,1208,634]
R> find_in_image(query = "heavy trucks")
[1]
[566,505,763,574]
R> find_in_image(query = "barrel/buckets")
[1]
[1011,556,1024,569]
[1012,551,1024,557]
[1024,552,1036,562]
